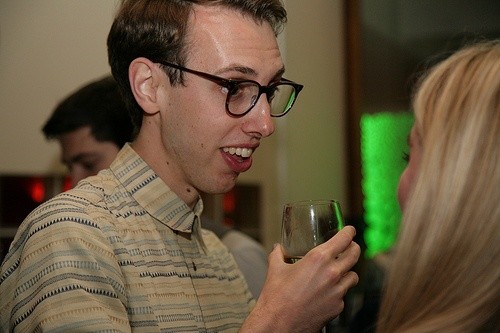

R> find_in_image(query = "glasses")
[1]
[150,58,304,117]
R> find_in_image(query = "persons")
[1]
[0,1,361,333]
[39,74,270,305]
[372,38,500,333]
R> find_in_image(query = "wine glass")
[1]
[280,200,349,333]
